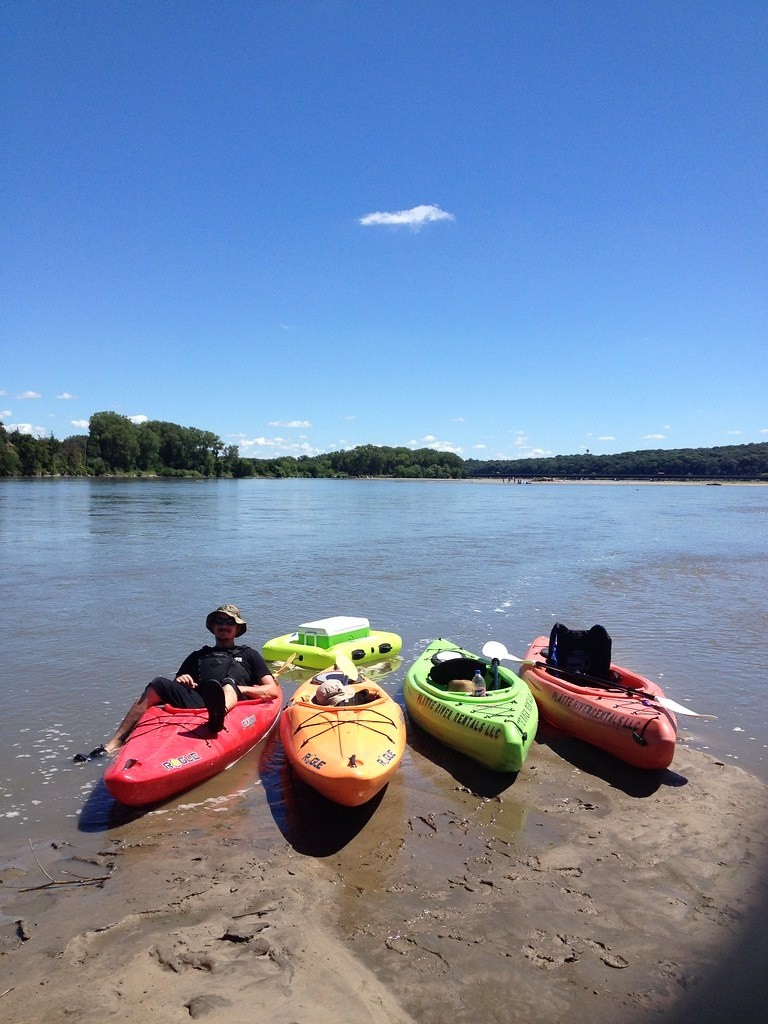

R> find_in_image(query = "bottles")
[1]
[473,669,486,697]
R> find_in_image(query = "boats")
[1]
[258,613,403,672]
[403,636,539,774]
[514,623,678,771]
[101,674,281,806]
[277,653,413,804]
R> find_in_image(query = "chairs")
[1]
[547,621,623,688]
[430,658,487,686]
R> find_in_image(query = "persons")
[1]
[72,605,279,764]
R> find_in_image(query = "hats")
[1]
[316,679,355,707]
[206,605,247,637]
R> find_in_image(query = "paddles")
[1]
[335,654,359,707]
[272,653,296,677]
[483,641,717,719]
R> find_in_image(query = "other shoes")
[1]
[74,744,108,762]
[203,679,226,732]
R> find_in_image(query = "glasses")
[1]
[213,617,235,626]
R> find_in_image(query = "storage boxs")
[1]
[298,616,369,650]
